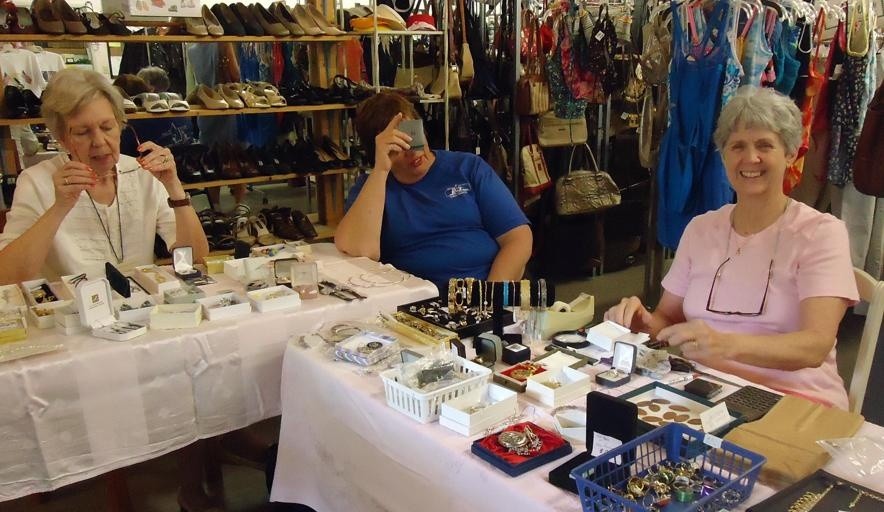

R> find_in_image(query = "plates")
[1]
[0,0,449,255]
[448,0,884,302]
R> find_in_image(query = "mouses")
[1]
[661,0,879,30]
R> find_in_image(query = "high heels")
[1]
[23,90,43,113]
[142,93,170,112]
[163,93,190,112]
[5,85,27,115]
[175,135,358,250]
[197,75,343,109]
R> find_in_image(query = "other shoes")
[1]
[2,2,132,37]
[185,2,343,36]
[121,97,137,112]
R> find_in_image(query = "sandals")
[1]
[195,203,318,250]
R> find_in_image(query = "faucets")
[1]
[195,203,318,250]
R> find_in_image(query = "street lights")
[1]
[366,35,462,105]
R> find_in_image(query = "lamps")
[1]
[848,266,884,415]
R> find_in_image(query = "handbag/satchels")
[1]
[450,3,668,216]
[366,35,462,105]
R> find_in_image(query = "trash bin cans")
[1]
[321,280,366,299]
[318,286,353,302]
[166,189,191,207]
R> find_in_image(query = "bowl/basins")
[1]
[731,199,787,256]
[84,167,131,273]
[446,278,547,323]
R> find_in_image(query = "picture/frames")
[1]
[0,242,441,511]
[269,302,884,512]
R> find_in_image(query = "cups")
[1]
[706,258,772,317]
[68,121,144,182]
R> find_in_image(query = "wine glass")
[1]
[475,356,483,363]
[607,367,617,378]
[64,177,70,185]
[410,300,491,330]
[591,460,741,511]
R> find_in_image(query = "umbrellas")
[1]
[175,135,358,250]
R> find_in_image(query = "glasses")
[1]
[68,121,144,182]
[706,258,772,317]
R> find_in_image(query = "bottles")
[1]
[337,4,437,32]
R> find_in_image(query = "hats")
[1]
[337,4,437,32]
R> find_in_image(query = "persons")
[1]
[334,92,535,284]
[602,85,860,414]
[0,69,210,285]
